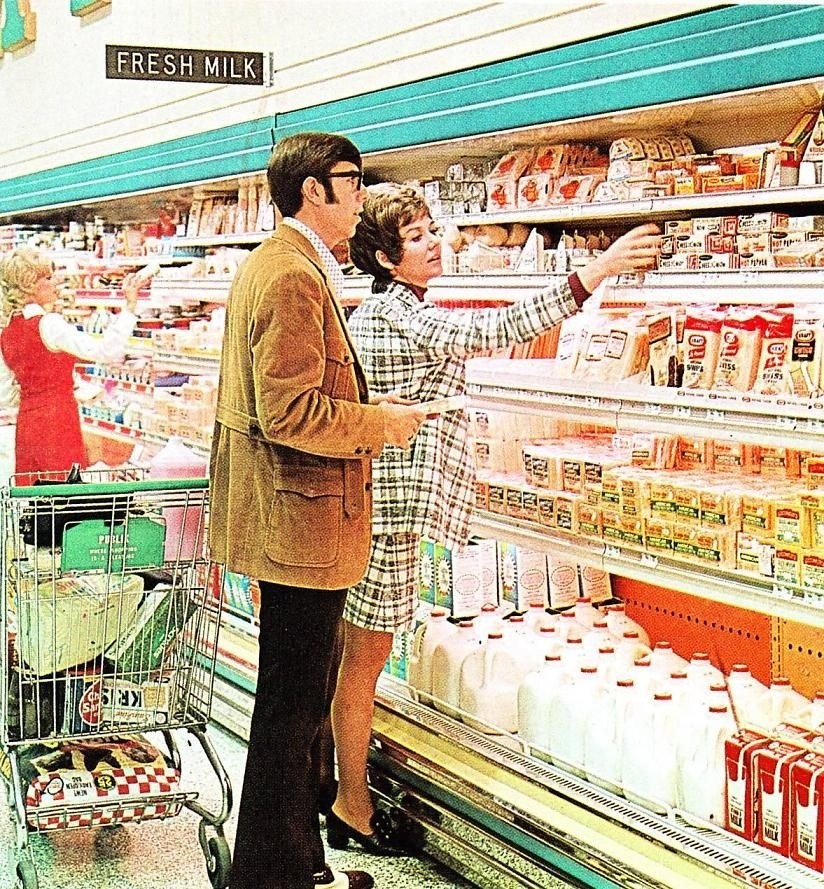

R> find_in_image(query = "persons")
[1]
[0,246,147,487]
[321,187,666,855]
[208,132,438,889]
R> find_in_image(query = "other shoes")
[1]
[313,865,374,889]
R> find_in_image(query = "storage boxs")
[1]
[4,520,197,730]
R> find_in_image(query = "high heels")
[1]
[316,781,341,816]
[325,805,422,858]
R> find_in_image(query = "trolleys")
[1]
[1,458,234,889]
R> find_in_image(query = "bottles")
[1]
[5,666,50,739]
[405,594,824,828]
[147,435,207,561]
[14,215,155,260]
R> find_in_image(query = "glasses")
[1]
[319,170,363,191]
